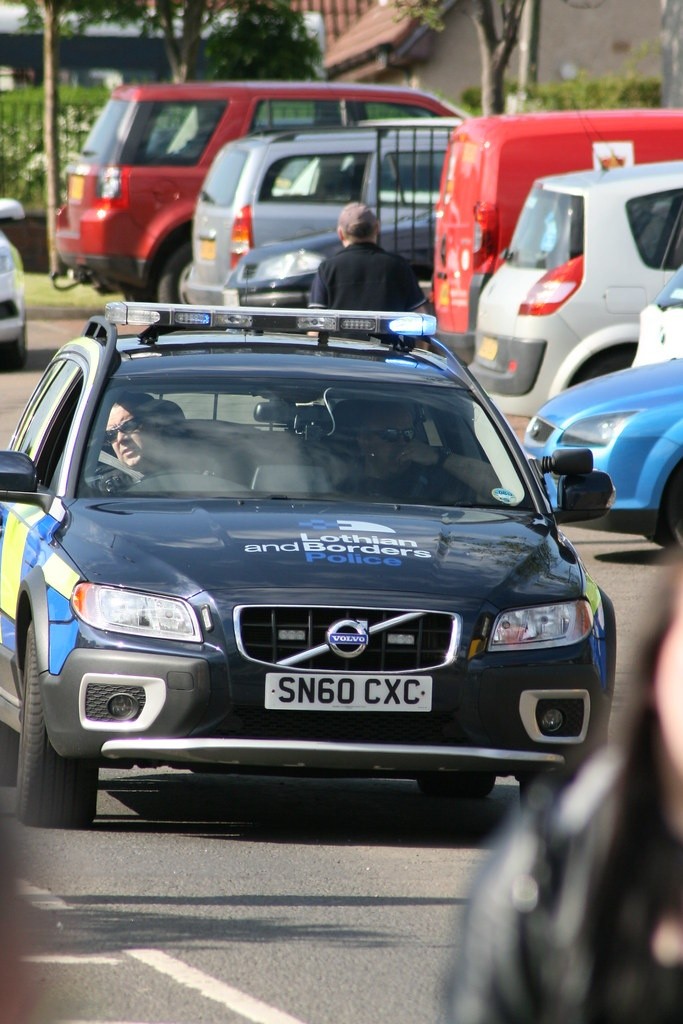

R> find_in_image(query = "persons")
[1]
[85,393,223,498]
[332,399,506,510]
[305,201,429,354]
[438,526,682,1024]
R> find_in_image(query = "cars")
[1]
[0,196,32,368]
[633,263,683,374]
[464,156,683,411]
[47,78,472,303]
[520,356,682,573]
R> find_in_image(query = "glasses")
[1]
[103,417,141,443]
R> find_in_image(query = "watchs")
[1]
[433,444,453,472]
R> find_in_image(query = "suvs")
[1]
[0,298,619,830]
[176,118,468,306]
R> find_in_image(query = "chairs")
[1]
[321,399,382,465]
[152,400,239,487]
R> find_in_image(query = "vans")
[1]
[428,109,683,333]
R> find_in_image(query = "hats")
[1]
[341,200,376,240]
